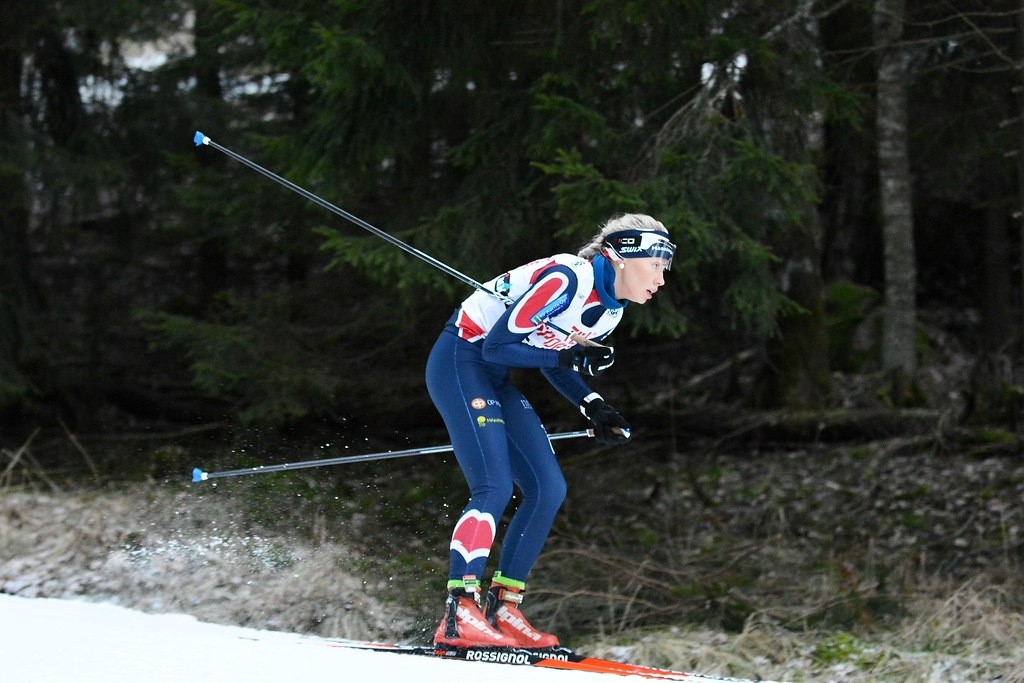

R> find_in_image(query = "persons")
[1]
[426,214,676,650]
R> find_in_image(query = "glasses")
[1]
[639,237,676,272]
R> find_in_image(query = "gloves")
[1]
[560,338,616,376]
[585,398,633,446]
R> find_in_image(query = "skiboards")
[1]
[319,635,766,682]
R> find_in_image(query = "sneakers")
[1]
[433,595,518,658]
[483,588,560,650]
